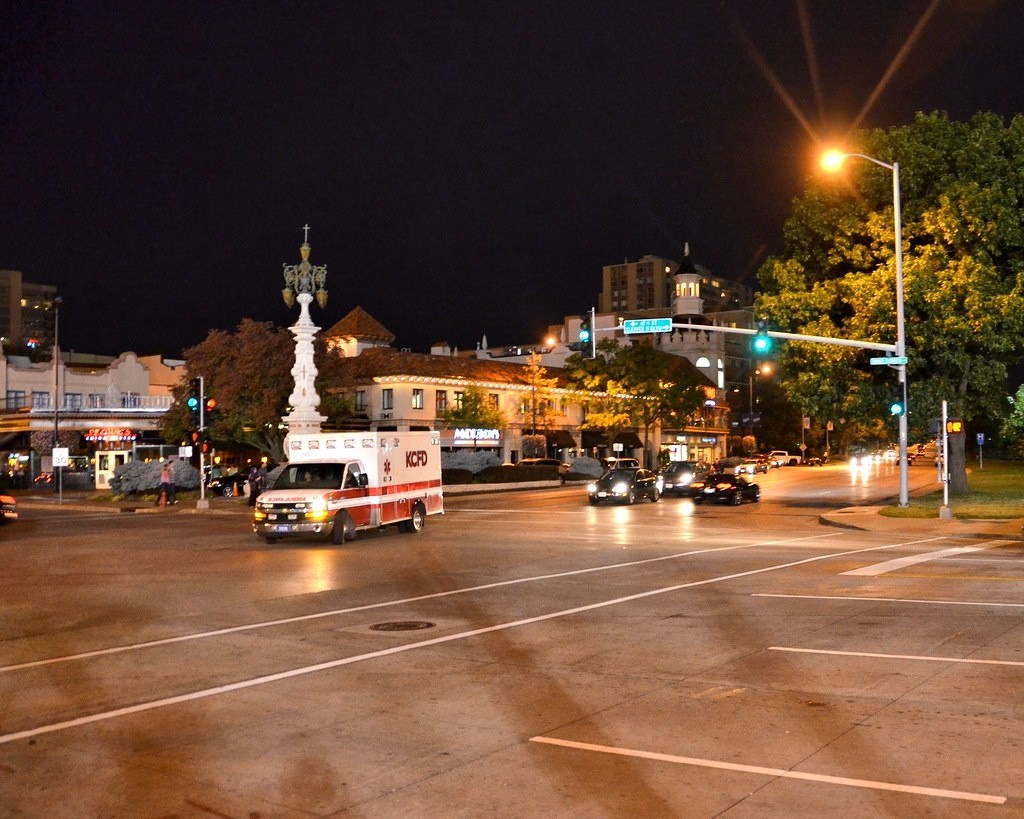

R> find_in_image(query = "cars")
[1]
[717,455,780,470]
[604,457,651,476]
[932,452,945,467]
[663,460,714,495]
[883,444,926,467]
[586,466,664,505]
[0,488,17,523]
[803,455,828,467]
[695,472,761,506]
[734,460,770,475]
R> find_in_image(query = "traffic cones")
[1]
[231,482,240,497]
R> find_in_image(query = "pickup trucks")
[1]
[764,450,803,470]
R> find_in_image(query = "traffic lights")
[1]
[203,397,216,429]
[189,431,201,443]
[580,307,599,360]
[890,403,905,416]
[750,318,771,355]
[200,442,212,454]
[186,377,199,411]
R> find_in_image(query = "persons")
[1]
[154,460,179,506]
[248,467,261,508]
[342,470,355,488]
[300,471,313,484]
[257,461,269,495]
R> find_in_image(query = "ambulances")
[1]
[250,428,447,544]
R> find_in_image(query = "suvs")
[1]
[522,459,572,485]
[206,463,280,498]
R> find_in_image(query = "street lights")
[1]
[17,297,60,491]
[817,146,917,509]
